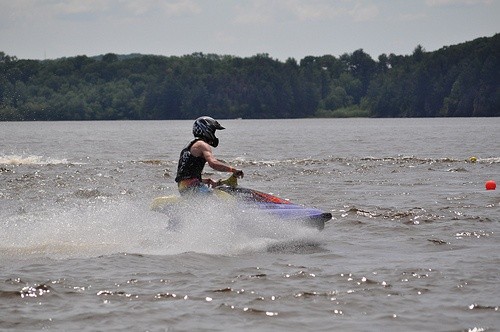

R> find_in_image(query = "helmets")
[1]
[192,116,225,148]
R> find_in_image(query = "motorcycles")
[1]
[151,169,333,235]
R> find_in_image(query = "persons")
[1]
[176,115,244,202]
